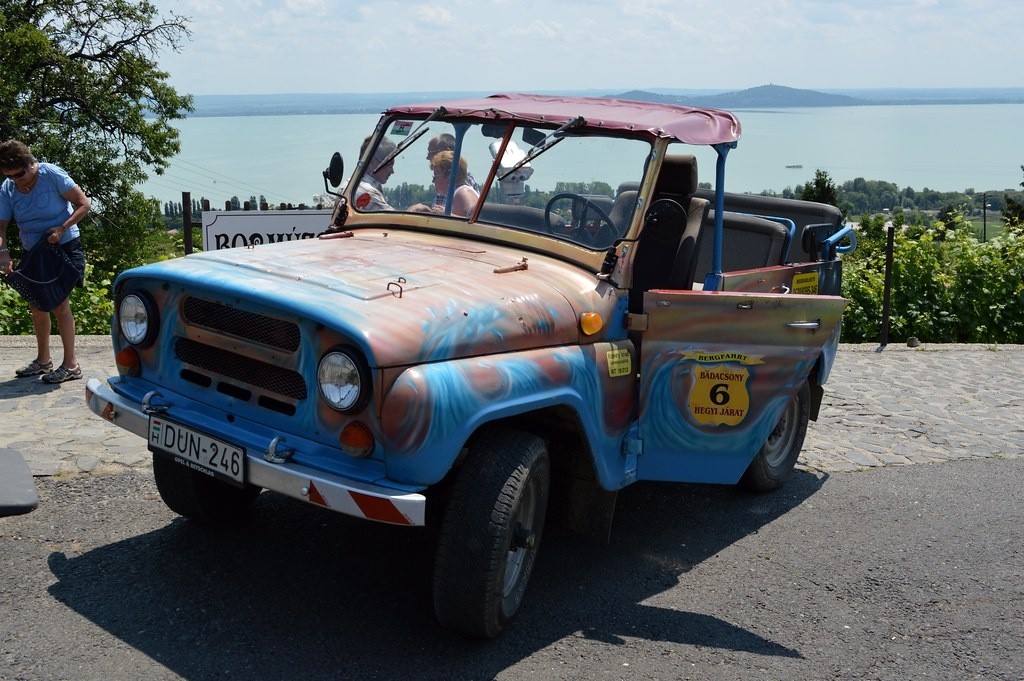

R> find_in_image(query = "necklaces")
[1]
[19,172,37,193]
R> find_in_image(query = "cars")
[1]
[84,93,858,640]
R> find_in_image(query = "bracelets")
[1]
[0,249,10,253]
[62,224,68,231]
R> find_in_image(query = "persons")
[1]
[426,133,480,218]
[0,140,91,384]
[334,134,432,212]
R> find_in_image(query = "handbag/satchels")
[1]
[0,233,80,312]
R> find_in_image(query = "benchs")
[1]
[601,191,797,290]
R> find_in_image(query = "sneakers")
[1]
[15,357,54,376]
[42,362,83,383]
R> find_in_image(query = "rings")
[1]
[0,265,5,270]
[51,233,56,238]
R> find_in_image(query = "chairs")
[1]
[595,152,711,354]
[466,203,566,236]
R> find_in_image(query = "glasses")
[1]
[426,149,442,155]
[6,167,26,179]
[431,170,451,178]
[373,156,395,168]
